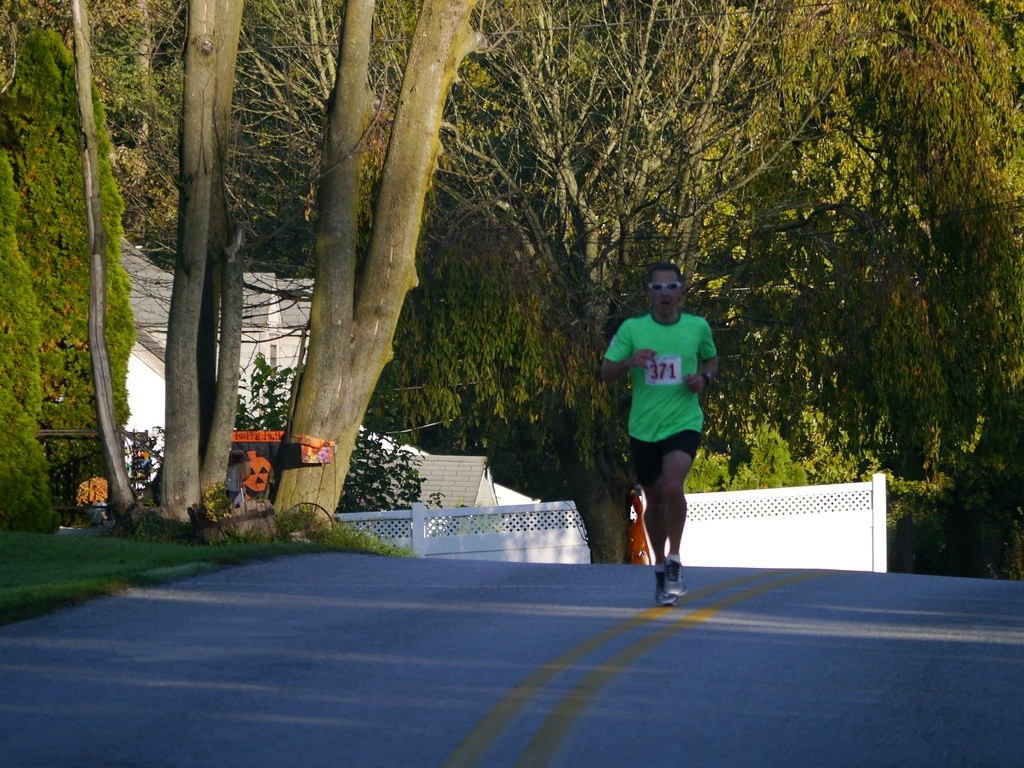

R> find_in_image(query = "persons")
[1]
[600,262,718,606]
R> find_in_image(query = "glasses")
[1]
[648,282,682,290]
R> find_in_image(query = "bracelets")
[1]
[699,371,712,387]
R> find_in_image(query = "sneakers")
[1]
[662,560,689,596]
[654,570,680,606]
